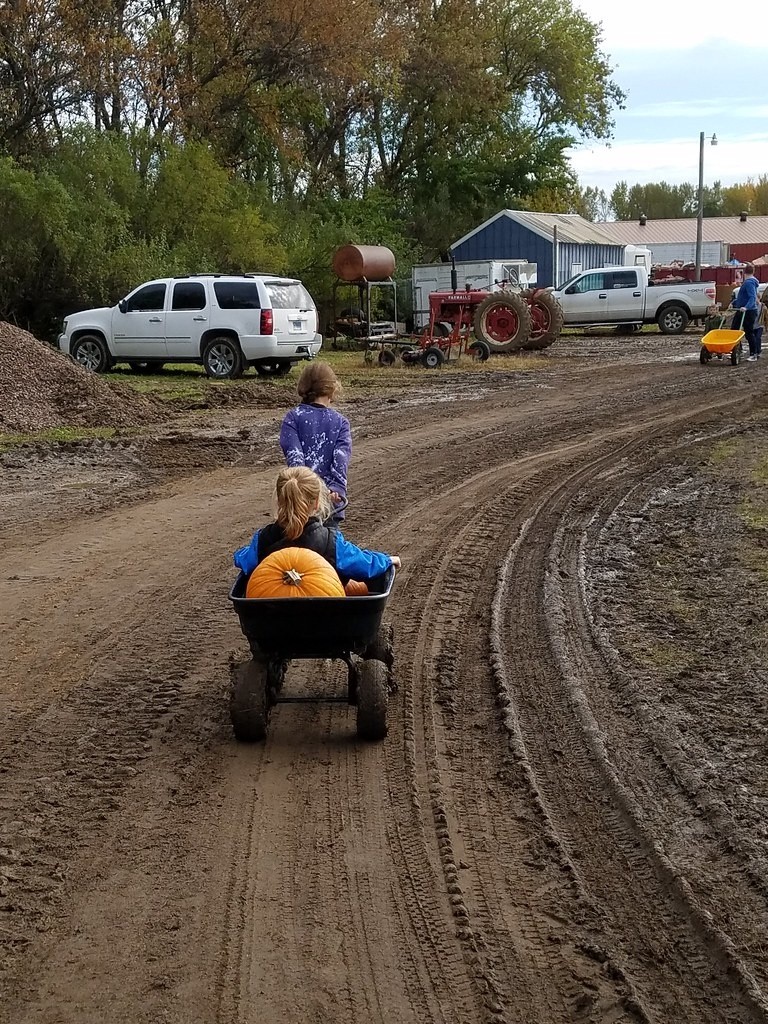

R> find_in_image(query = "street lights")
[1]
[694,132,718,325]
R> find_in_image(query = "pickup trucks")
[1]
[528,266,717,335]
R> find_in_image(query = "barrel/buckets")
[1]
[332,245,395,281]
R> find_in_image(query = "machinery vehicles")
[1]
[419,255,565,354]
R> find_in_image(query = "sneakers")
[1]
[726,352,744,359]
[745,354,757,361]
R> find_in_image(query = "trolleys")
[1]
[699,308,746,365]
[229,495,396,744]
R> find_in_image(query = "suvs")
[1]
[57,271,318,380]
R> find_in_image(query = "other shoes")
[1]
[756,353,761,357]
[718,359,723,362]
[708,359,713,361]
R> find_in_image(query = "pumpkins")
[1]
[343,579,368,596]
[246,547,345,597]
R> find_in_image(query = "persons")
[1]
[281,360,352,527]
[703,304,728,361]
[728,264,768,362]
[233,466,402,592]
